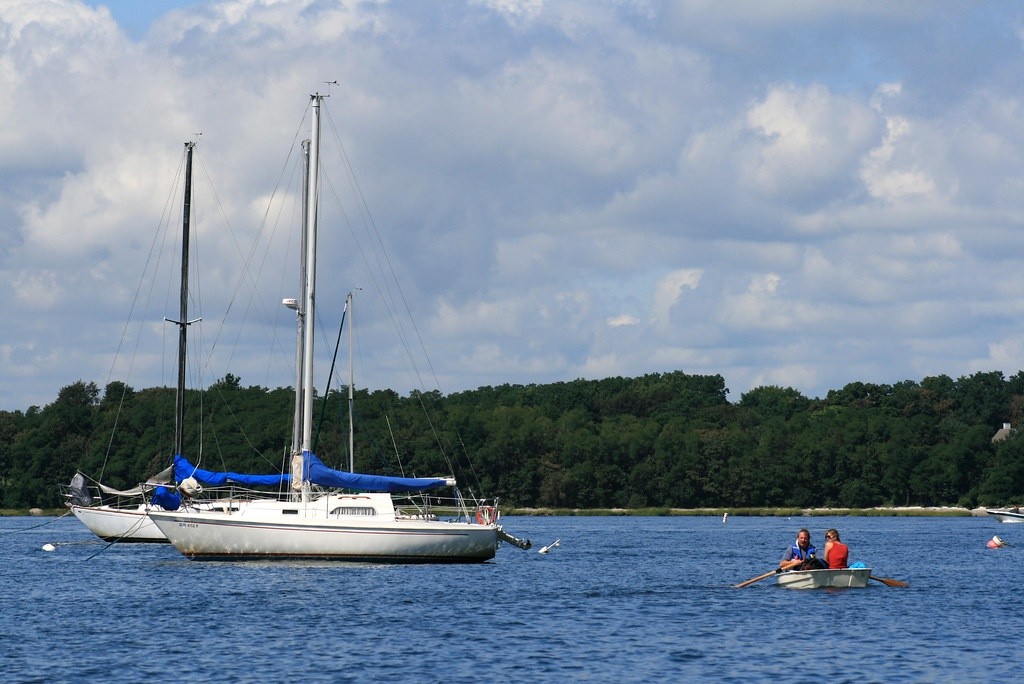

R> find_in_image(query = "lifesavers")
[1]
[477,506,497,525]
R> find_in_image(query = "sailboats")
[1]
[54,81,534,562]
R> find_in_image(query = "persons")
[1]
[1014,505,1019,514]
[779,529,816,571]
[824,528,848,569]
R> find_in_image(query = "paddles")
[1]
[735,561,804,588]
[870,575,907,587]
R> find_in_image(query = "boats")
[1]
[988,509,1024,525]
[775,566,873,590]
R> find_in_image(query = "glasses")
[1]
[825,535,835,539]
